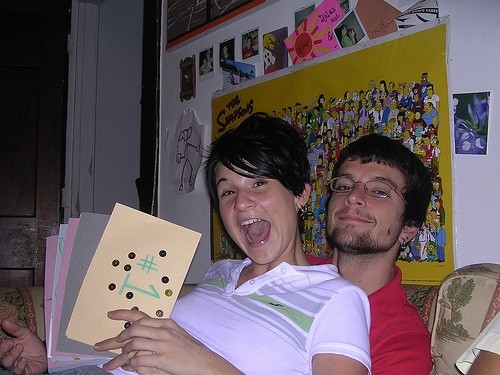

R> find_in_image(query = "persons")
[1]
[339,25,357,48]
[455,311,500,375]
[0,114,433,375]
[200,36,256,74]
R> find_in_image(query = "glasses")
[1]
[330,177,408,204]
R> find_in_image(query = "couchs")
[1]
[0,262,500,375]
